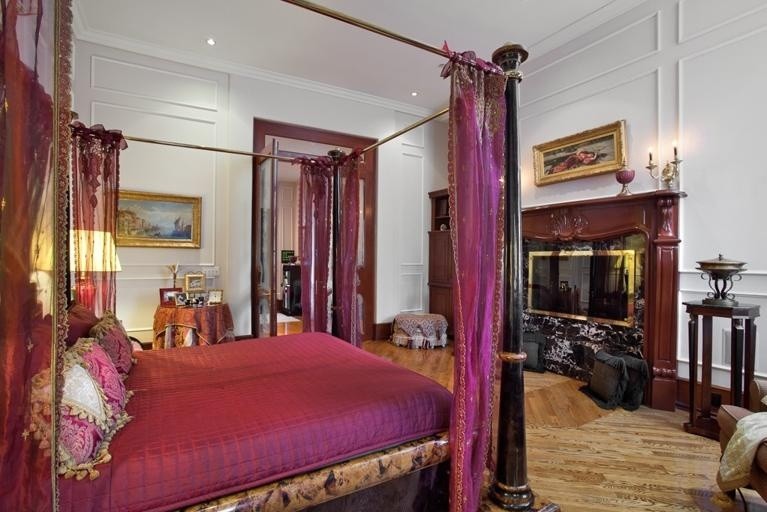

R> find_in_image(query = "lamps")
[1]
[68,230,122,313]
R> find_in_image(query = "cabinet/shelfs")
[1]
[427,187,455,338]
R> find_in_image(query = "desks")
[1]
[154,302,231,350]
[681,298,760,444]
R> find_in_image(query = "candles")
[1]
[649,153,653,166]
[673,147,678,160]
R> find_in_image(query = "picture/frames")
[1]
[159,274,223,308]
[532,119,627,187]
[116,189,203,250]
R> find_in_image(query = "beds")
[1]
[3,293,458,506]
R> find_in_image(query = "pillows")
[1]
[22,299,136,483]
[579,351,650,412]
[521,332,547,374]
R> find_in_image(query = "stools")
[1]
[393,314,449,350]
